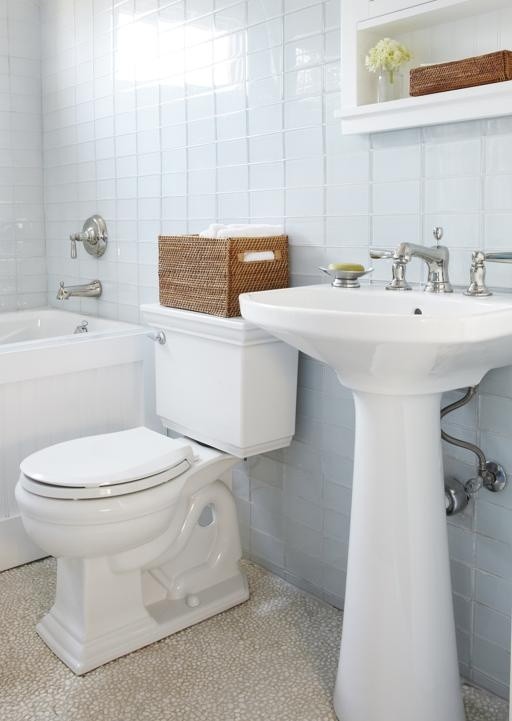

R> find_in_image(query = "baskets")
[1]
[158,234,289,318]
[410,50,512,96]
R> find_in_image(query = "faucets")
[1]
[393,241,455,293]
[55,279,102,302]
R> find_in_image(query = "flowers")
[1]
[363,38,414,85]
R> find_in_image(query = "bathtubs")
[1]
[1,304,169,578]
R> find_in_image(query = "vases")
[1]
[375,68,404,104]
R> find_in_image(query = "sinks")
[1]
[238,279,511,397]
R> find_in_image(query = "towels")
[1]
[198,223,285,263]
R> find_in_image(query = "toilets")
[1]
[12,301,300,682]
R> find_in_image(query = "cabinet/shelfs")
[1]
[333,0,512,136]
